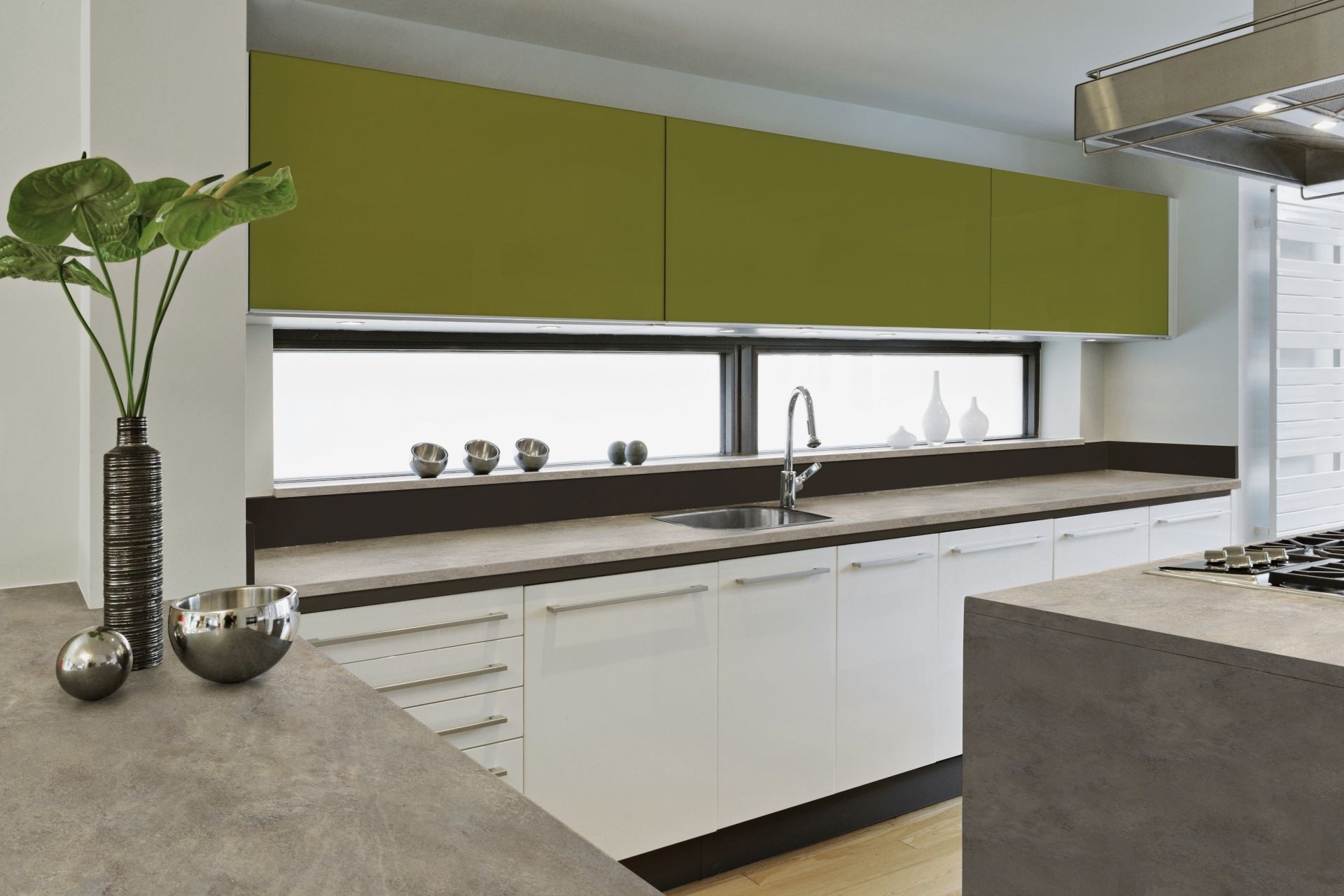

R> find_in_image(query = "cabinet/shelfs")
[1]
[249,49,1169,342]
[299,494,1236,872]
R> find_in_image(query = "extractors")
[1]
[1074,0,1344,200]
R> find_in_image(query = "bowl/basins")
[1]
[409,442,449,477]
[513,437,550,471]
[168,584,301,684]
[462,439,500,474]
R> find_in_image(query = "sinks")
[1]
[650,502,835,531]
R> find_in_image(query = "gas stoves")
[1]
[1142,528,1344,599]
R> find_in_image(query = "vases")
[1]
[104,415,164,671]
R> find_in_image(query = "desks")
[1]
[0,583,666,896]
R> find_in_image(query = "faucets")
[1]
[779,386,822,509]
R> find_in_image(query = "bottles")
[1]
[922,371,951,447]
[959,396,989,444]
[886,426,917,449]
